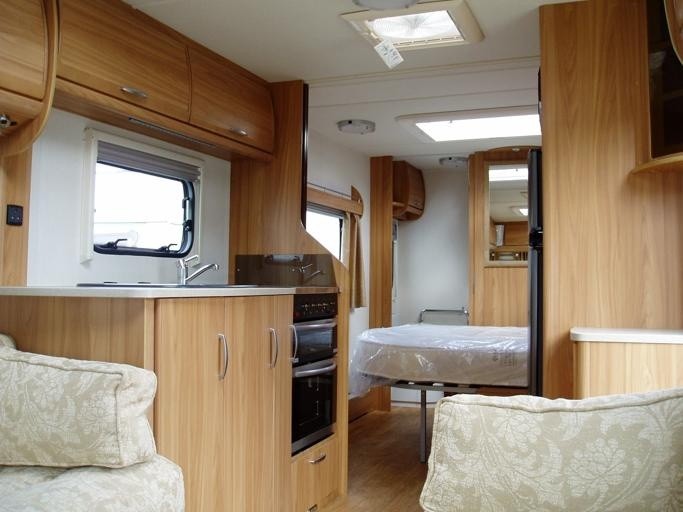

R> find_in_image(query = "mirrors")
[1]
[483,158,528,268]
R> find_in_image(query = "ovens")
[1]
[290,317,338,455]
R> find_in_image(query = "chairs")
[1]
[418,387,683,512]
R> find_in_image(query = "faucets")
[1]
[175,254,220,285]
[300,264,327,286]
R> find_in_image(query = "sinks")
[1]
[76,280,259,288]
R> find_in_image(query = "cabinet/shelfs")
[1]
[632,0,683,174]
[392,161,425,221]
[52,0,276,163]
[0,0,49,100]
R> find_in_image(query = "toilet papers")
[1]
[271,254,304,264]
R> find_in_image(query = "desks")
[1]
[352,323,529,463]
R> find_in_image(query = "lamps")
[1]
[352,0,418,11]
[439,157,468,169]
[337,119,375,135]
